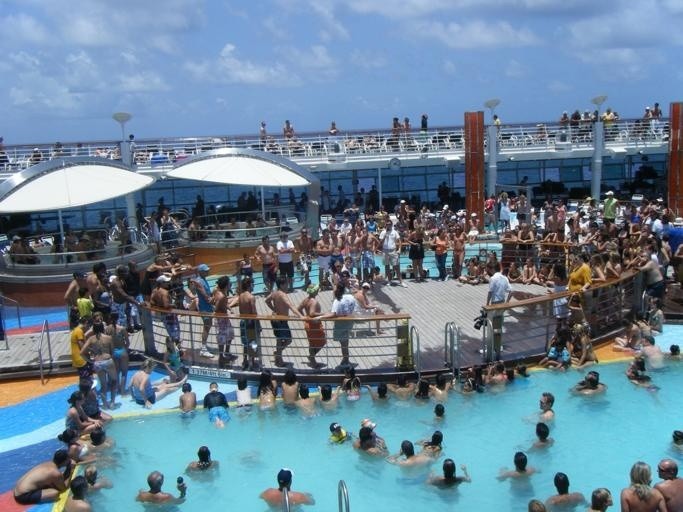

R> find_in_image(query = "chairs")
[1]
[328,275,338,303]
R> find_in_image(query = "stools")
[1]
[351,321,369,338]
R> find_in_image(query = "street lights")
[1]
[112,112,136,239]
[484,97,500,197]
[590,95,606,201]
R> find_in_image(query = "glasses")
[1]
[657,465,668,473]
[364,287,370,290]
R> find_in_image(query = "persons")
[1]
[259,120,305,154]
[536,123,546,139]
[0,136,10,168]
[391,117,411,148]
[51,141,64,156]
[494,114,500,128]
[635,102,662,135]
[129,134,137,152]
[560,109,619,143]
[93,147,112,157]
[29,148,43,165]
[1,176,682,512]
[328,121,339,136]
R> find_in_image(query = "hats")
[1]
[329,423,341,432]
[443,204,449,210]
[585,196,594,201]
[400,199,406,203]
[604,190,614,196]
[196,263,211,272]
[156,275,171,282]
[361,418,376,431]
[362,282,370,288]
[673,217,683,225]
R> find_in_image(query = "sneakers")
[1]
[164,349,359,370]
